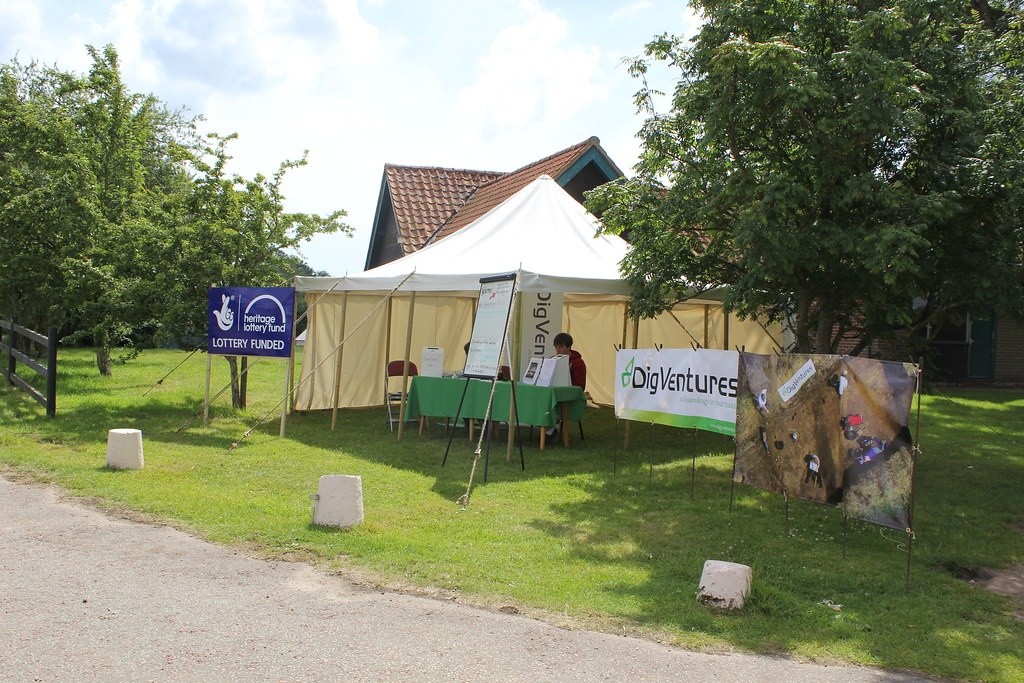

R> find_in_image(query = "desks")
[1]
[403,376,587,449]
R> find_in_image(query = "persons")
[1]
[758,389,768,417]
[538,332,586,444]
[841,414,864,431]
[805,455,823,488]
[831,374,847,395]
[856,440,886,464]
[462,341,489,439]
[761,427,770,455]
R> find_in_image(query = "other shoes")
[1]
[539,428,558,445]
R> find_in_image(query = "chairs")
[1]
[499,366,585,443]
[385,360,430,432]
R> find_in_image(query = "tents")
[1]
[279,174,796,463]
